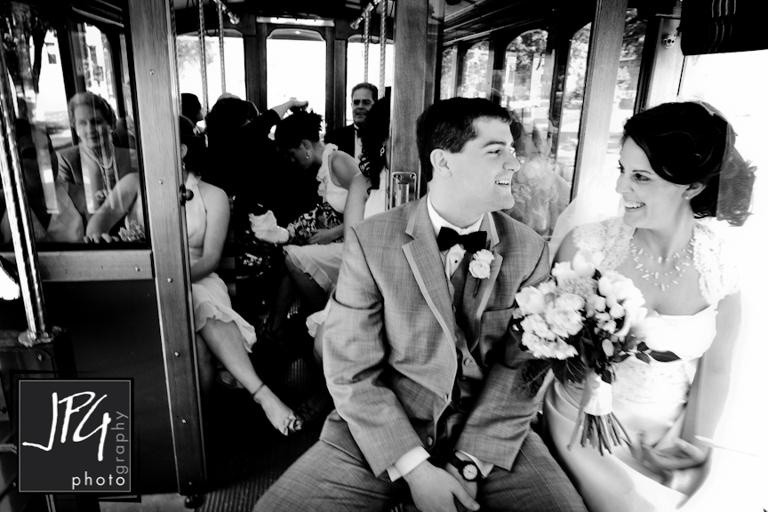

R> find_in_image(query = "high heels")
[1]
[253,382,303,437]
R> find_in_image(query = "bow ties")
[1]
[436,226,487,253]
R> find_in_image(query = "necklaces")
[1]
[626,234,695,294]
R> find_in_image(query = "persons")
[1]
[0,84,586,512]
[548,99,757,511]
